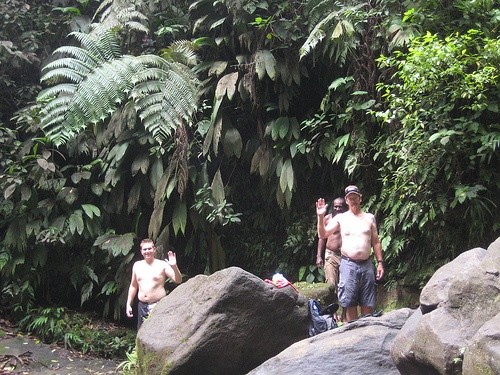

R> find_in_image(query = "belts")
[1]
[342,256,371,264]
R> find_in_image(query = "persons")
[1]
[315,185,385,322]
[316,197,347,323]
[125,238,182,332]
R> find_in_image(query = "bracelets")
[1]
[377,260,384,264]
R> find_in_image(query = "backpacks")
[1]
[307,298,339,336]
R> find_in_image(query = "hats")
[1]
[344,185,362,198]
